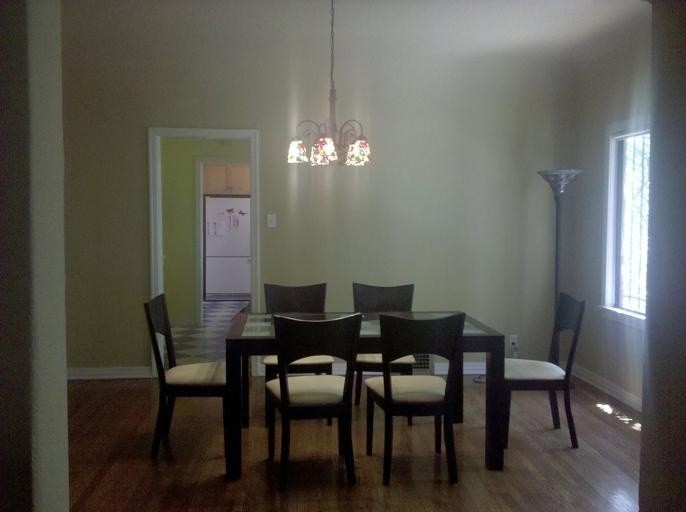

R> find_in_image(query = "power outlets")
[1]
[509,335,518,354]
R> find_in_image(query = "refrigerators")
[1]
[205,193,252,302]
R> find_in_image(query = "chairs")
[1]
[351,281,418,406]
[262,283,334,431]
[364,312,468,486]
[142,290,228,460]
[261,313,361,487]
[499,290,585,448]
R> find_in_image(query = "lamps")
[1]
[534,169,583,389]
[286,1,373,168]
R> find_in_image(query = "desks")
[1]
[224,310,508,479]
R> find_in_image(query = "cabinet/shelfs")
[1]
[203,162,250,195]
